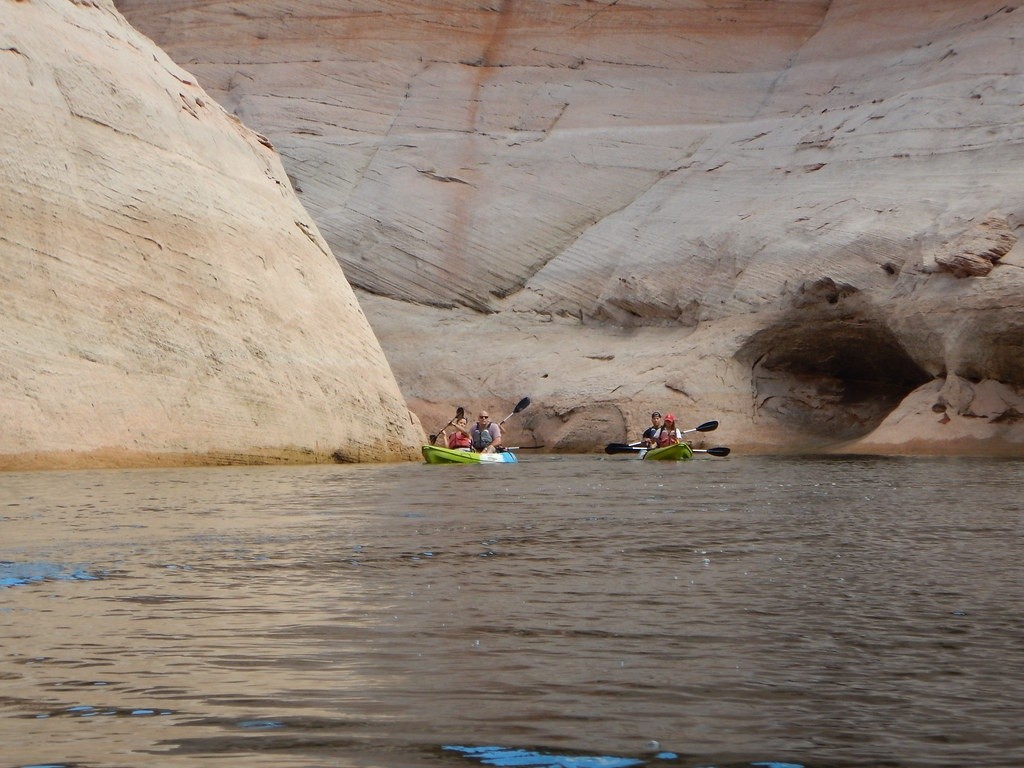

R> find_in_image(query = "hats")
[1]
[652,411,662,418]
[665,413,675,422]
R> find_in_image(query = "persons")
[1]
[651,414,682,448]
[643,412,662,447]
[470,411,501,454]
[439,417,474,452]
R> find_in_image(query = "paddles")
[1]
[499,445,545,452]
[497,397,532,426]
[604,420,731,458]
[429,407,465,447]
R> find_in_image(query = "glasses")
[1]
[480,416,488,418]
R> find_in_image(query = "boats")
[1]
[637,440,693,459]
[420,443,519,464]
